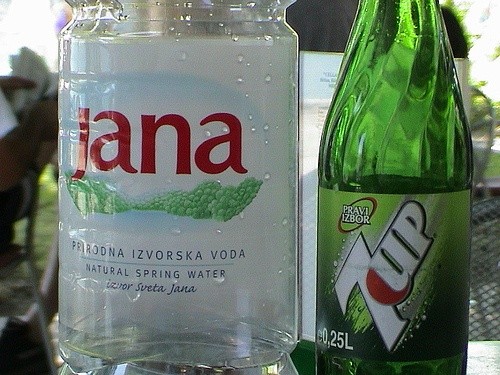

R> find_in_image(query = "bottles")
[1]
[52,0,299,371]
[317,0,474,375]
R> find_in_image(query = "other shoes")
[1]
[1,315,53,364]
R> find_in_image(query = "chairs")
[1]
[461,85,497,340]
[0,96,60,375]
[464,192,500,341]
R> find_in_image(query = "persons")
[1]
[26,224,61,335]
[0,75,34,268]
